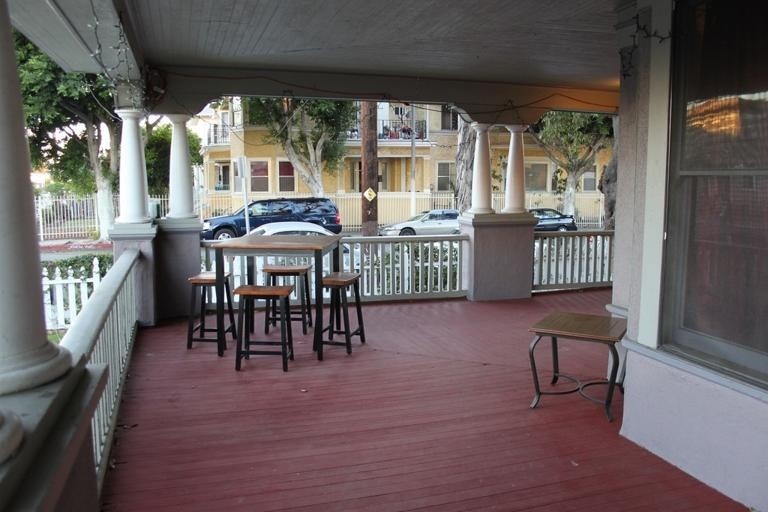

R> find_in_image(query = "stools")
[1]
[232,285,295,372]
[263,264,313,336]
[186,271,237,350]
[527,309,627,422]
[313,271,365,355]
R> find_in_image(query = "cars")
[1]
[210,221,362,273]
[377,210,461,236]
[200,196,342,248]
[528,208,577,232]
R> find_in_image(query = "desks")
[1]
[210,235,344,356]
